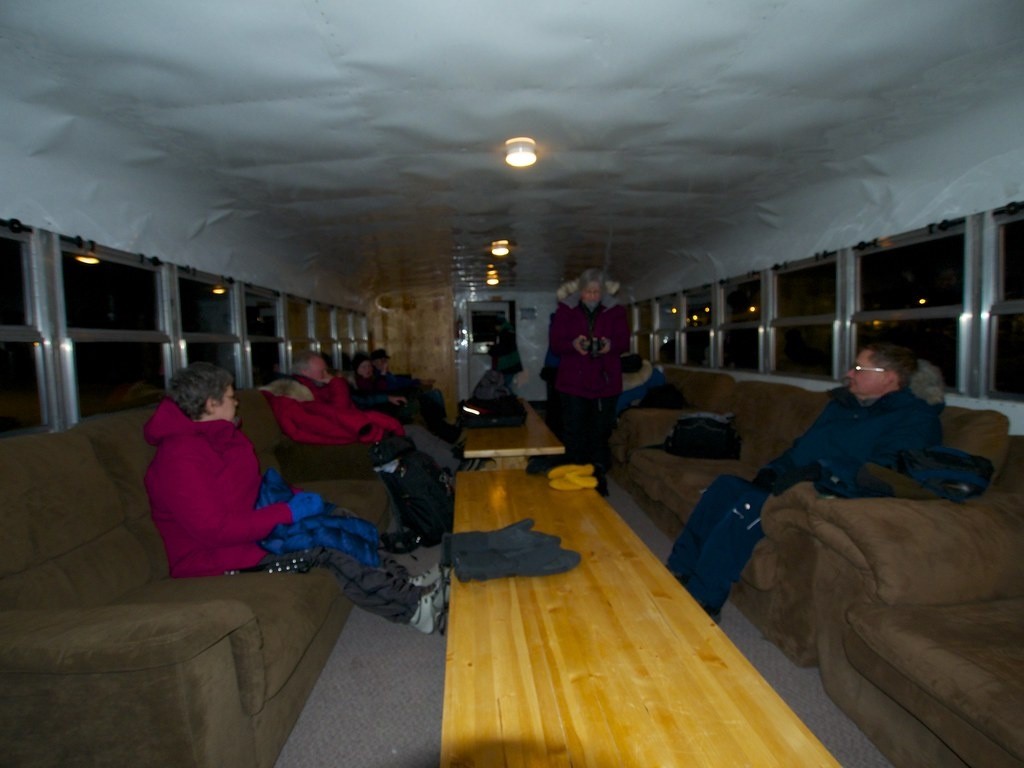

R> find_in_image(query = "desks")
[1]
[440,472,843,768]
[463,397,565,472]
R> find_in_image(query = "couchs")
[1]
[606,368,736,492]
[624,380,1024,670]
[808,492,1024,768]
[0,388,394,768]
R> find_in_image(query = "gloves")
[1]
[773,463,820,496]
[440,518,582,582]
[547,463,599,491]
[752,468,777,491]
[289,491,325,523]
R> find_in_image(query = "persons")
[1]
[668,345,947,625]
[487,314,524,388]
[542,268,632,497]
[258,349,481,473]
[145,361,450,634]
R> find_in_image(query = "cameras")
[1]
[583,336,605,358]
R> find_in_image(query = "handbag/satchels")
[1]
[540,366,558,380]
[462,393,527,428]
[638,383,693,408]
[899,445,996,503]
[496,349,520,371]
[621,354,642,373]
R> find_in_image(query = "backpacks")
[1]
[369,436,455,553]
[646,412,743,460]
[474,369,510,400]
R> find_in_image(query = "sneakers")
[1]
[408,566,451,636]
[408,562,450,587]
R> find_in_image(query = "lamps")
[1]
[491,240,509,255]
[506,137,538,167]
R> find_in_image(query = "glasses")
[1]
[849,361,886,373]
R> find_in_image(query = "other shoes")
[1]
[596,478,608,496]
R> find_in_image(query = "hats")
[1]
[371,349,390,361]
[352,352,370,370]
